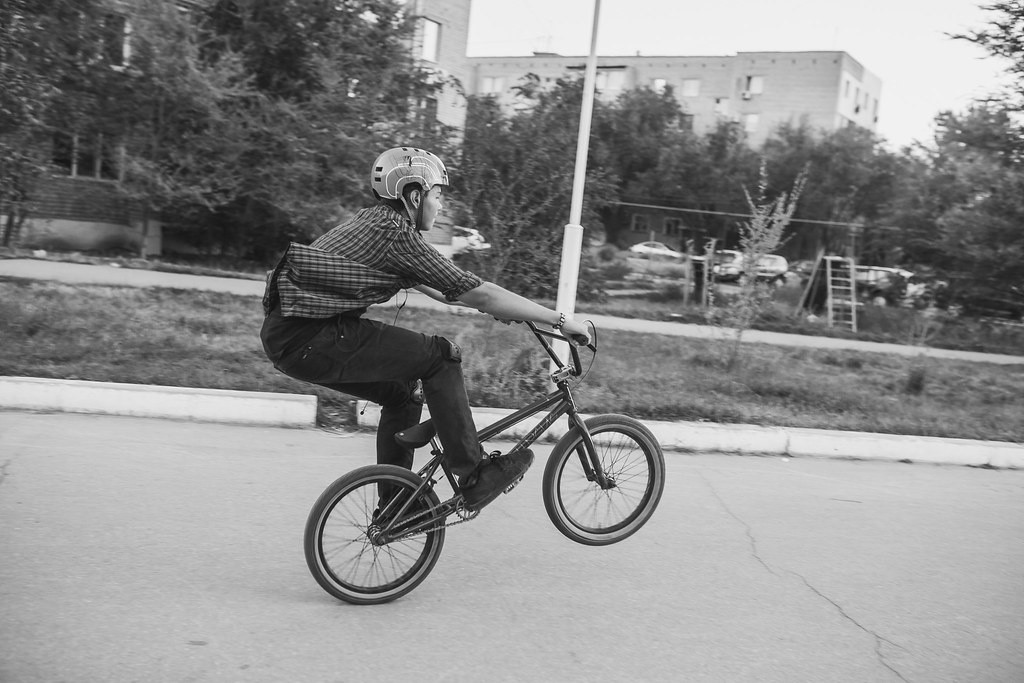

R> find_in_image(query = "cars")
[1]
[430,225,492,259]
[712,249,925,307]
[933,277,1024,323]
[628,241,684,258]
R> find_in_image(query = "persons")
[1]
[261,147,594,525]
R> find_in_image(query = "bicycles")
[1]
[303,319,666,606]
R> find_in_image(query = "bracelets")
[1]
[552,313,565,328]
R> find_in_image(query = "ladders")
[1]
[825,255,859,334]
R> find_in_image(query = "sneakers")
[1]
[372,504,452,533]
[458,448,534,511]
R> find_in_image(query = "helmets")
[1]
[370,147,450,199]
